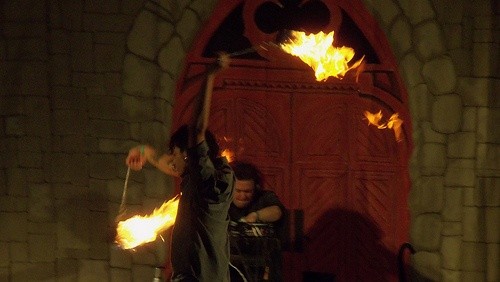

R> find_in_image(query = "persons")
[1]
[126,51,237,281]
[226,159,284,281]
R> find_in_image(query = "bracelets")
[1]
[140,145,145,159]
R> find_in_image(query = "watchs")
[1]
[254,211,261,224]
